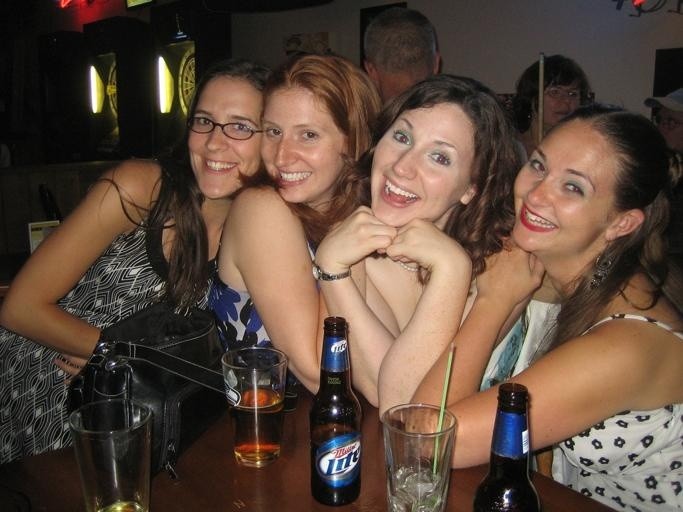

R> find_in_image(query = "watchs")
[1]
[311,261,351,282]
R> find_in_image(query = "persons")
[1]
[512,54,591,158]
[398,103,682,511]
[310,74,522,429]
[643,87,682,157]
[1,59,278,474]
[361,7,442,104]
[205,51,385,402]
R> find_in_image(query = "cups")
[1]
[223,346,288,464]
[379,401,458,511]
[69,398,152,510]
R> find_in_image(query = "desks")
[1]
[0,376,624,511]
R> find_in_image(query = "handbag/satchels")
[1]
[67,302,228,501]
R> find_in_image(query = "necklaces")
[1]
[388,253,429,276]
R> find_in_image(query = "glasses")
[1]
[652,115,683,131]
[543,86,580,99]
[187,117,264,140]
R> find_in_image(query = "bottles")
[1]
[471,381,539,510]
[308,315,363,502]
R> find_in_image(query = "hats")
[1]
[643,87,683,112]
